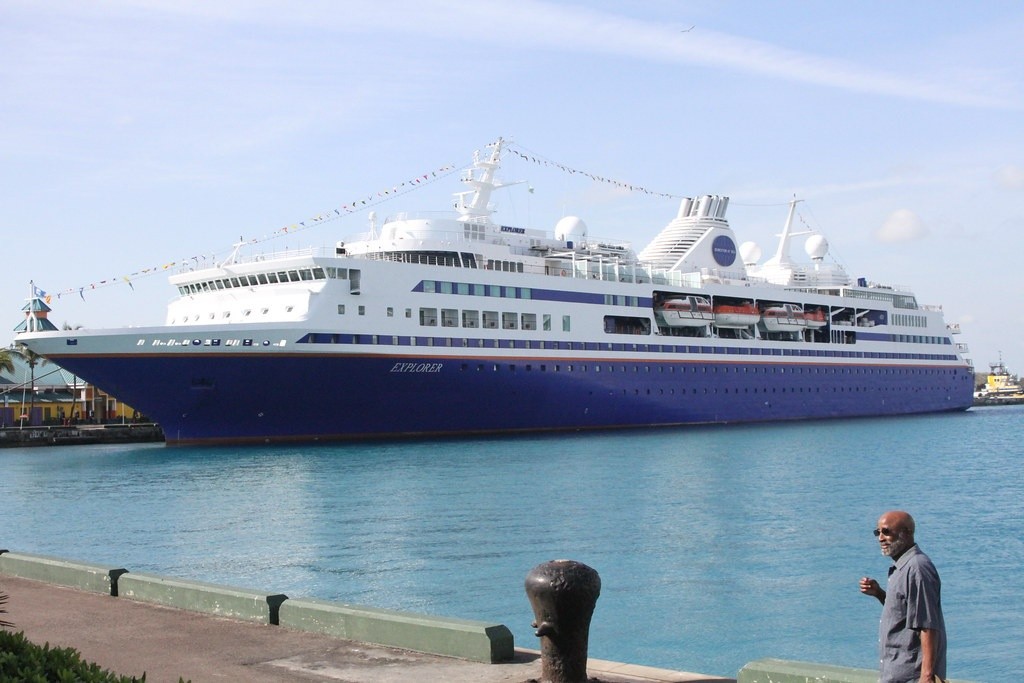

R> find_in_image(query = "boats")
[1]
[971,350,1024,407]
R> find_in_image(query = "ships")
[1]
[11,139,977,447]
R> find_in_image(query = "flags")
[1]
[34,286,46,298]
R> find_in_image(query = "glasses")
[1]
[873,528,895,536]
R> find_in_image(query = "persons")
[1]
[64,417,72,427]
[859,510,946,683]
[131,410,138,423]
